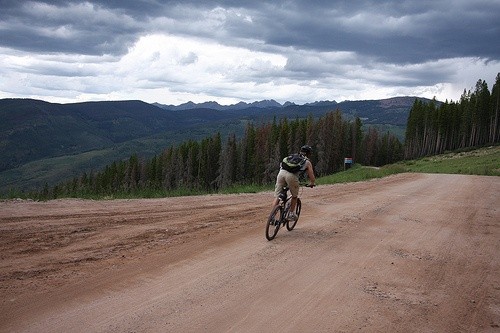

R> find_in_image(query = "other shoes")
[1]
[288,212,298,221]
[270,218,275,225]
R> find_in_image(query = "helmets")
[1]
[301,145,313,153]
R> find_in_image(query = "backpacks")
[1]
[282,153,306,173]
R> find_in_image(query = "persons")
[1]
[270,145,315,224]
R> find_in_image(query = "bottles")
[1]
[284,201,291,211]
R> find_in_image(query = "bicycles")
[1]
[265,182,317,240]
